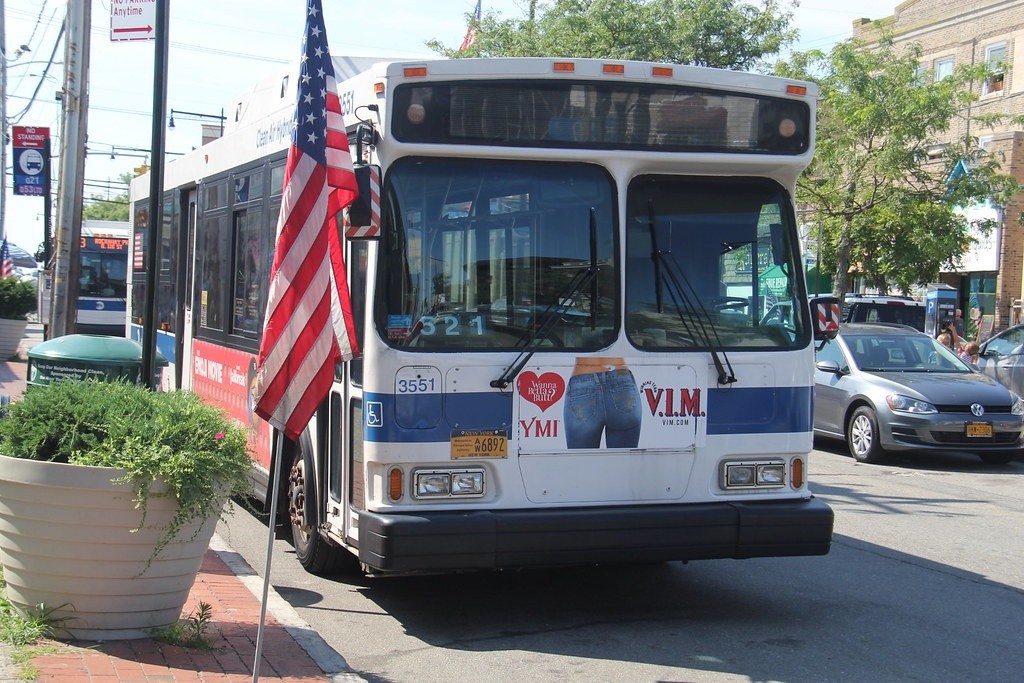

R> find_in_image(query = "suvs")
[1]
[759,292,926,364]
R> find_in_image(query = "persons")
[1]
[564,357,642,449]
[247,357,261,438]
[956,309,964,338]
[949,321,980,371]
[927,333,953,369]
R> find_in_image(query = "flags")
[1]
[254,0,361,442]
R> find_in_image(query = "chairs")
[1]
[866,346,889,368]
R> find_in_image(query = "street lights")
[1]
[168,107,227,137]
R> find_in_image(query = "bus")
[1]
[38,220,129,341]
[126,54,840,581]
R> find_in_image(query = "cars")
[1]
[787,322,1024,463]
[974,323,1024,400]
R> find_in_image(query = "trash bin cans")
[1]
[25,332,168,396]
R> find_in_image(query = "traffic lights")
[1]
[133,165,146,177]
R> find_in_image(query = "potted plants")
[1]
[1,369,267,641]
